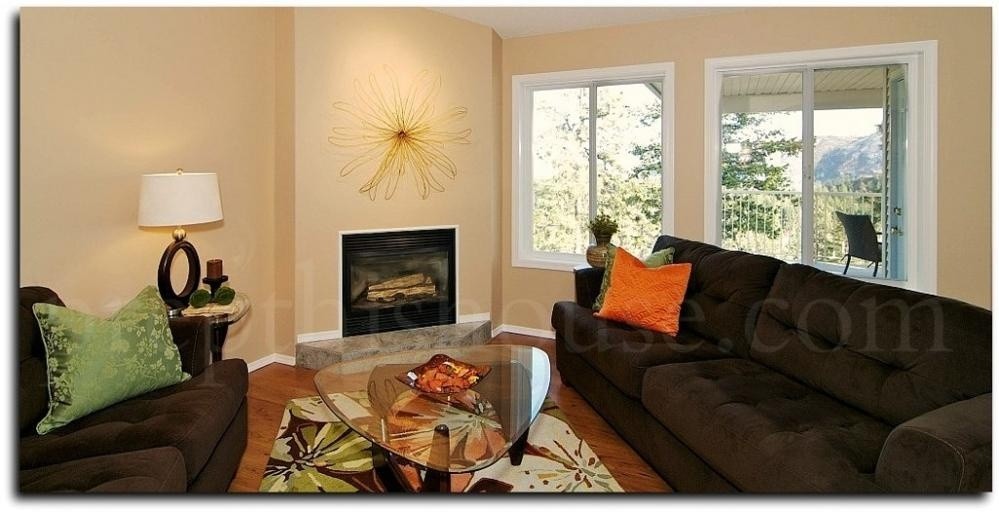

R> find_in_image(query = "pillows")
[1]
[592,246,692,337]
[31,285,192,436]
[591,242,675,312]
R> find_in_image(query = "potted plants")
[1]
[584,211,620,268]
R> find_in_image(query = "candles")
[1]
[206,258,222,279]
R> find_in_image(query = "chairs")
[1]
[835,211,883,278]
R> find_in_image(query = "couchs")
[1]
[19,285,249,496]
[547,233,992,493]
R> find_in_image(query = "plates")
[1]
[395,353,491,395]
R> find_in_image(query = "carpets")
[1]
[258,389,625,492]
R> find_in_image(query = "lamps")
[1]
[136,167,223,316]
